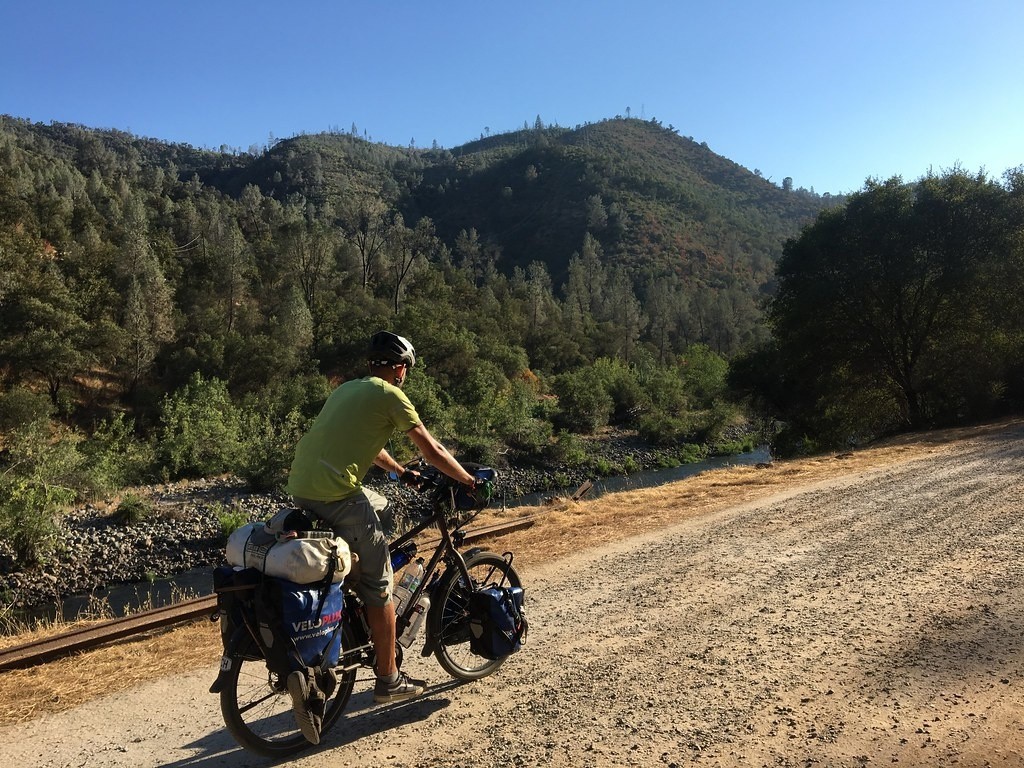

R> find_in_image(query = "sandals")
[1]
[287,668,337,744]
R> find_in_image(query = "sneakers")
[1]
[373,671,428,703]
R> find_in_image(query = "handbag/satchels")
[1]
[225,520,353,585]
[212,565,343,680]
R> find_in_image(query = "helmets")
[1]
[370,331,417,368]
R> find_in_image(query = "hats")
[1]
[250,508,313,547]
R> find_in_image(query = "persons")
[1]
[286,330,479,702]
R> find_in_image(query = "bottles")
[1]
[352,595,371,631]
[390,542,417,574]
[393,557,424,616]
[397,586,430,649]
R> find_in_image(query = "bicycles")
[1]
[209,456,524,759]
